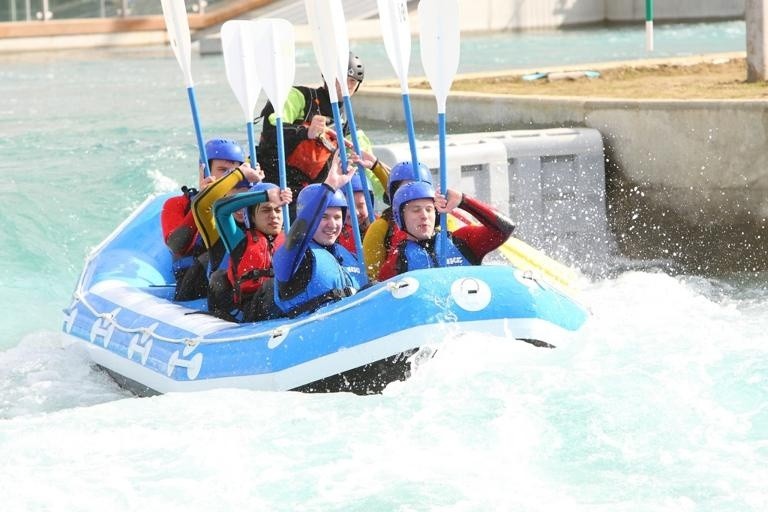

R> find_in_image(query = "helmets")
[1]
[341,173,375,211]
[391,180,441,231]
[386,160,435,209]
[295,182,348,233]
[346,51,364,93]
[200,137,246,178]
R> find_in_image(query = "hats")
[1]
[243,182,284,231]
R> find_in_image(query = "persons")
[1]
[190,162,266,316]
[257,51,391,224]
[269,148,361,319]
[362,162,462,282]
[336,172,381,255]
[374,180,515,281]
[212,182,293,320]
[161,138,245,302]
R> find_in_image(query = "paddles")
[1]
[161,0,210,178]
[304,120,584,289]
[377,0,420,180]
[420,0,459,267]
[221,18,256,184]
[306,0,375,222]
[253,19,295,233]
[310,1,366,283]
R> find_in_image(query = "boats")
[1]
[64,192,588,402]
